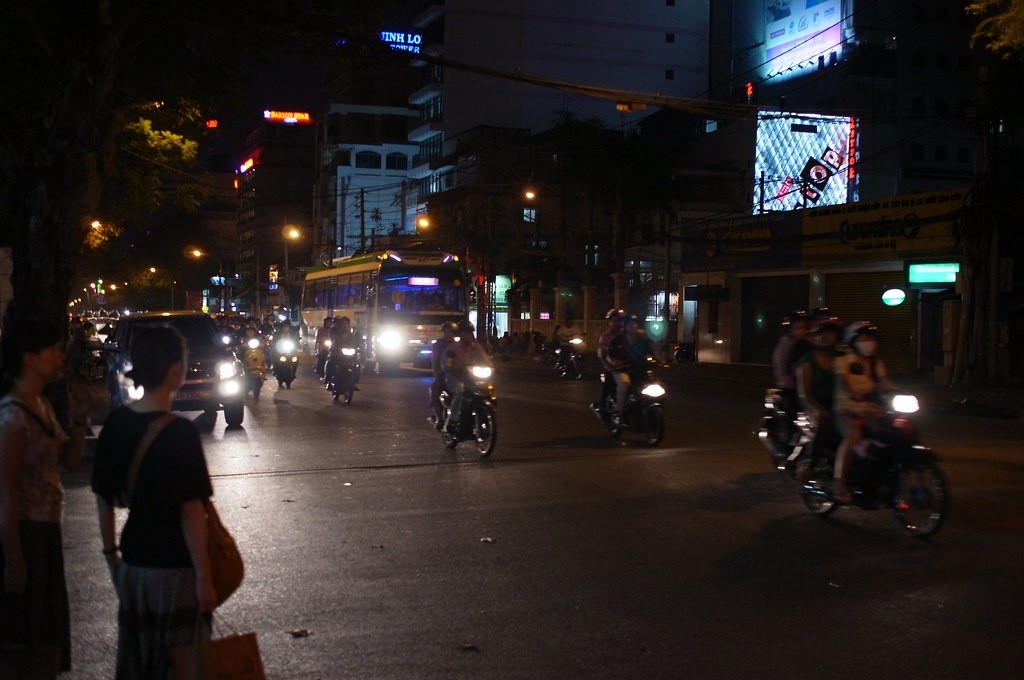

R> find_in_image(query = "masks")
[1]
[855,341,877,356]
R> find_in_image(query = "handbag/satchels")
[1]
[173,608,266,680]
[204,502,244,605]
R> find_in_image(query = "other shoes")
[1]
[325,382,331,390]
[434,418,443,429]
[831,477,851,501]
[443,422,453,432]
[794,459,813,479]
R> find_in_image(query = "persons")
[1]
[91,324,217,679]
[772,306,893,501]
[218,313,361,392]
[0,312,73,680]
[596,309,657,425]
[427,320,493,432]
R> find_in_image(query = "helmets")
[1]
[779,310,807,330]
[625,313,639,323]
[844,320,878,343]
[441,321,459,332]
[605,308,626,319]
[455,320,475,332]
[808,308,844,328]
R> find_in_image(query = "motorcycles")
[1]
[752,362,952,539]
[223,326,373,406]
[590,342,668,448]
[425,349,512,457]
[528,325,588,382]
[672,339,695,363]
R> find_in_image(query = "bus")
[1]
[299,249,486,375]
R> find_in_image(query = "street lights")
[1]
[418,215,471,294]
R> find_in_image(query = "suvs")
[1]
[100,310,246,426]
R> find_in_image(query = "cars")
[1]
[68,306,121,362]
